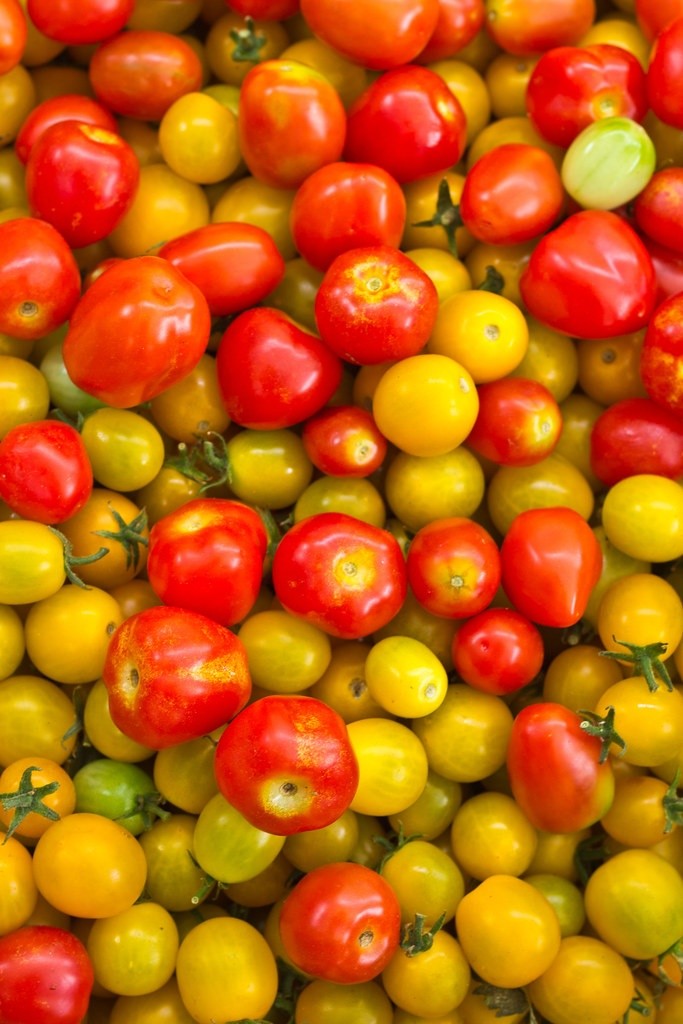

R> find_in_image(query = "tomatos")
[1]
[0,0,682,1024]
